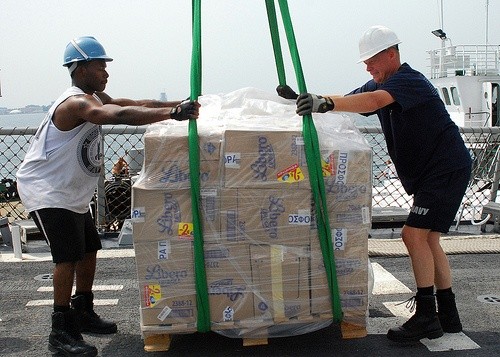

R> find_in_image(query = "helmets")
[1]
[356,25,401,63]
[62,36,113,67]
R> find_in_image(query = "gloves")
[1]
[276,84,299,99]
[296,93,334,116]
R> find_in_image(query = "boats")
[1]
[425,0,500,191]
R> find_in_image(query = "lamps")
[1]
[432,29,447,40]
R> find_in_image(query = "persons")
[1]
[276,27,472,342]
[103,161,130,228]
[17,35,203,357]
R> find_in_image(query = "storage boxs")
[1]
[131,130,373,325]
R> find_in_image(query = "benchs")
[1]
[8,220,39,258]
[371,206,409,229]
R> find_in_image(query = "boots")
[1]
[434,294,463,334]
[70,293,118,337]
[48,311,98,357]
[387,292,444,343]
